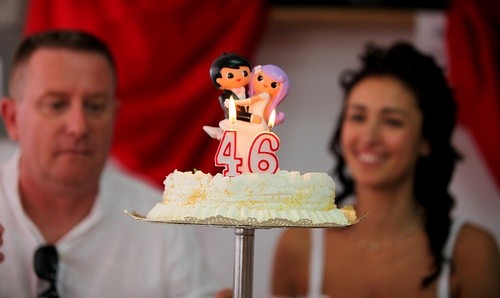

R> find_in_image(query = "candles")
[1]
[215,95,242,176]
[249,108,281,174]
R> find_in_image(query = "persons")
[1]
[0,29,232,298]
[209,50,262,125]
[271,39,500,298]
[203,65,291,141]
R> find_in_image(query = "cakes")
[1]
[145,49,357,225]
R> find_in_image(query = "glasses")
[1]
[33,244,61,298]
